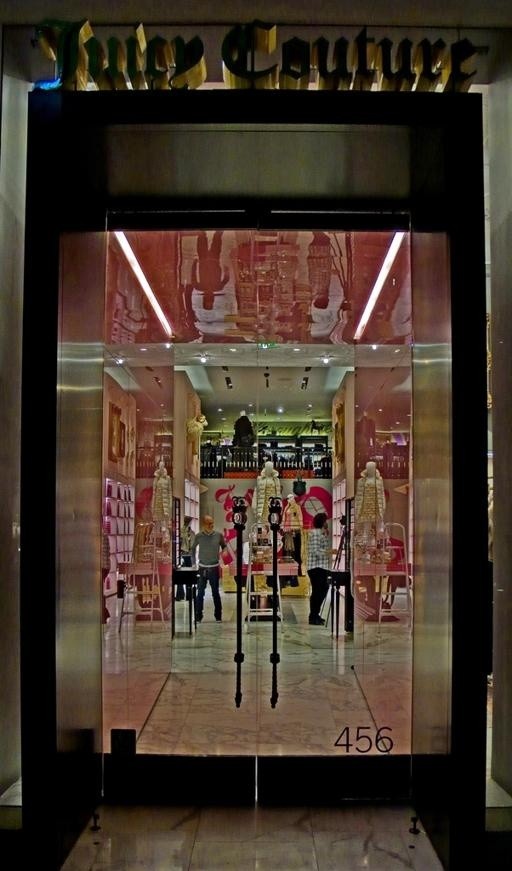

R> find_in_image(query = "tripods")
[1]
[319,532,347,628]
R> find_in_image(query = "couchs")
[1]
[222,565,310,597]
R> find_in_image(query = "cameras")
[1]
[338,515,347,525]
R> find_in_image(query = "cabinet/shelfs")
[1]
[184,477,200,563]
[328,570,350,640]
[103,477,135,598]
[331,479,347,599]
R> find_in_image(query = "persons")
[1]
[252,461,281,523]
[191,515,227,624]
[307,513,339,625]
[355,461,386,552]
[150,461,173,521]
[189,231,231,310]
[281,494,307,577]
[306,231,335,308]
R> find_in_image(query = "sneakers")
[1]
[215,615,221,623]
[194,614,203,623]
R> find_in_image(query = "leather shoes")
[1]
[318,616,325,621]
[309,619,324,625]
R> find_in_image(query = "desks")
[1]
[172,567,200,640]
